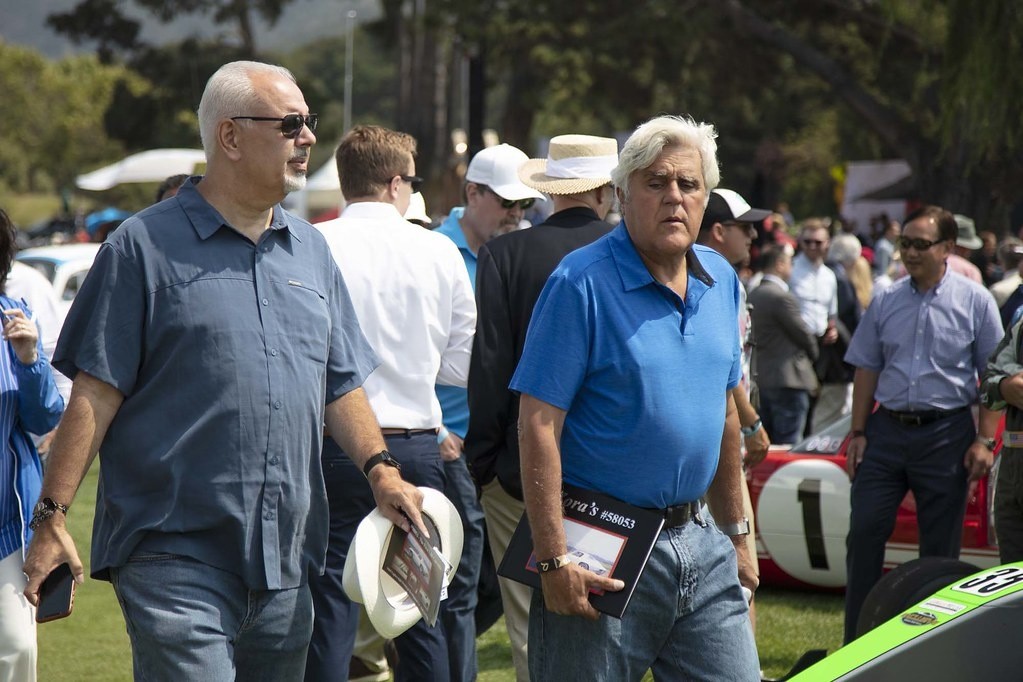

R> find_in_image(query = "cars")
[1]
[741,403,1007,595]
[14,242,105,326]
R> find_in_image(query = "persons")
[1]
[312,125,620,682]
[694,188,1023,648]
[507,115,760,682]
[23,60,429,682]
[0,174,194,682]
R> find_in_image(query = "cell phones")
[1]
[36,563,75,622]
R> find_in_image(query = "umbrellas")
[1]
[75,148,345,223]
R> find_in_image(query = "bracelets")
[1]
[850,431,865,439]
[536,555,571,574]
[29,497,68,532]
[741,415,762,436]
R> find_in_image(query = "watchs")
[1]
[976,434,997,450]
[362,450,401,479]
[716,516,750,536]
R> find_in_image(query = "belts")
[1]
[322,426,424,436]
[879,405,972,426]
[664,501,695,530]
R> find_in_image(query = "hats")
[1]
[953,214,984,249]
[466,143,547,201]
[700,188,772,230]
[342,487,464,640]
[518,134,619,195]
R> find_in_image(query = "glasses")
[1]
[800,240,827,246]
[899,236,948,250]
[479,185,536,209]
[230,113,319,138]
[386,175,424,193]
